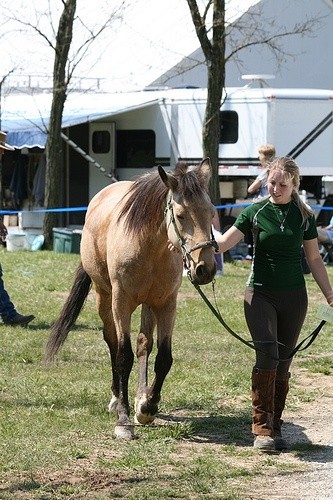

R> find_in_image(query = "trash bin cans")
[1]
[51,226,83,254]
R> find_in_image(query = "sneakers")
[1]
[275,436,288,449]
[253,435,276,451]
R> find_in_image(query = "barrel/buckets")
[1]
[7,233,25,250]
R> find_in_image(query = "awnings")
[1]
[0,98,162,149]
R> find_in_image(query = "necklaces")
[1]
[271,198,292,232]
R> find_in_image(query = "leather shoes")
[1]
[5,314,34,324]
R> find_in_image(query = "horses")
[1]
[41,157,214,440]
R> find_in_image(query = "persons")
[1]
[248,145,276,201]
[316,215,333,246]
[167,157,333,452]
[0,131,36,327]
